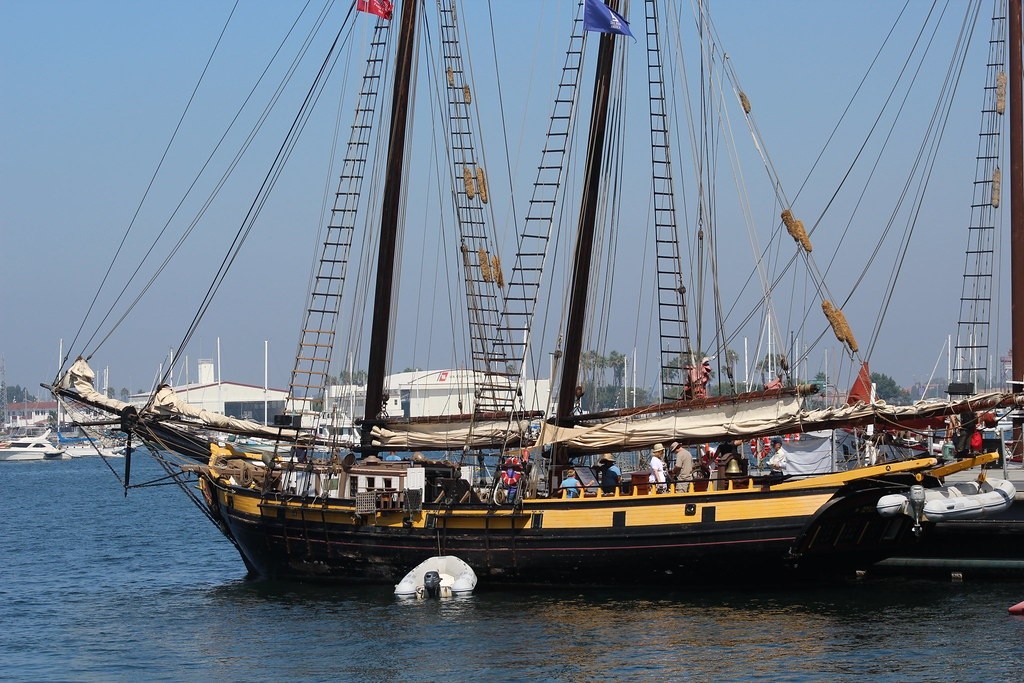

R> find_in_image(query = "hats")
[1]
[770,436,782,443]
[653,443,665,453]
[567,470,575,476]
[670,442,682,453]
[599,453,617,464]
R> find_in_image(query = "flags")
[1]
[357,0,394,21]
[582,0,637,44]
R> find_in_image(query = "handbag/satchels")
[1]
[970,430,982,450]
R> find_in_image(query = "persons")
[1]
[706,440,743,475]
[599,453,622,494]
[558,469,595,498]
[386,450,401,461]
[769,437,787,476]
[896,430,909,439]
[392,491,405,509]
[669,442,693,493]
[649,443,667,494]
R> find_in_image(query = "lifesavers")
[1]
[784,431,801,446]
[700,451,721,474]
[501,457,523,485]
[750,434,772,457]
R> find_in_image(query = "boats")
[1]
[0,433,69,461]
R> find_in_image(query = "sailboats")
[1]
[40,0,1023,592]
